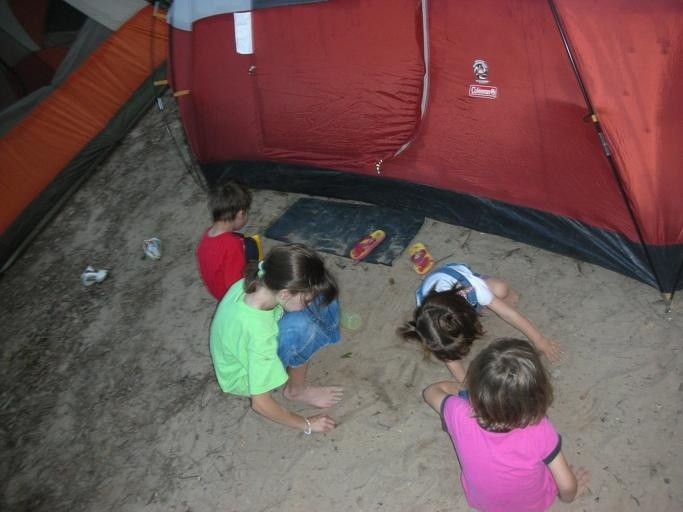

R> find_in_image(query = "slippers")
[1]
[351,229,385,261]
[408,243,434,275]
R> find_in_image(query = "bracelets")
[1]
[304,417,312,434]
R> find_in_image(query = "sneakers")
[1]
[143,238,162,260]
[80,265,109,286]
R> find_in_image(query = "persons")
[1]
[209,242,345,435]
[195,181,253,301]
[422,337,577,511]
[395,261,560,383]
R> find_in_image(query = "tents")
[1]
[147,2,681,312]
[1,1,170,271]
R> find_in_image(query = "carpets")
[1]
[262,197,424,267]
